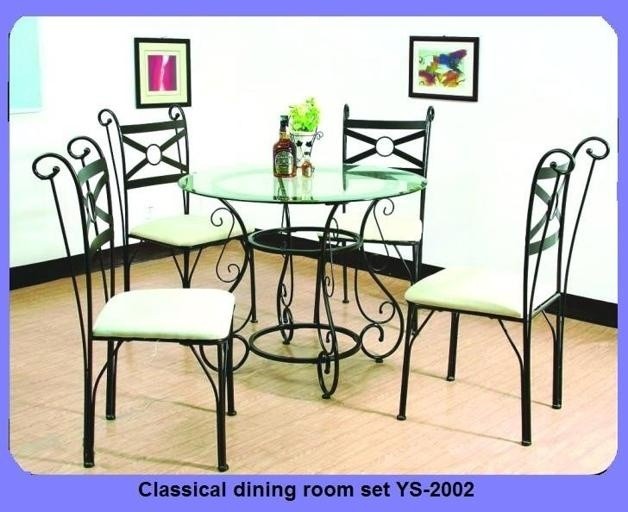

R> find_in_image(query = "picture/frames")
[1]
[134,38,192,109]
[408,36,479,103]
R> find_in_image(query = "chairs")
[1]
[32,101,611,473]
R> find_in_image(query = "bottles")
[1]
[272,120,298,179]
[302,158,313,177]
